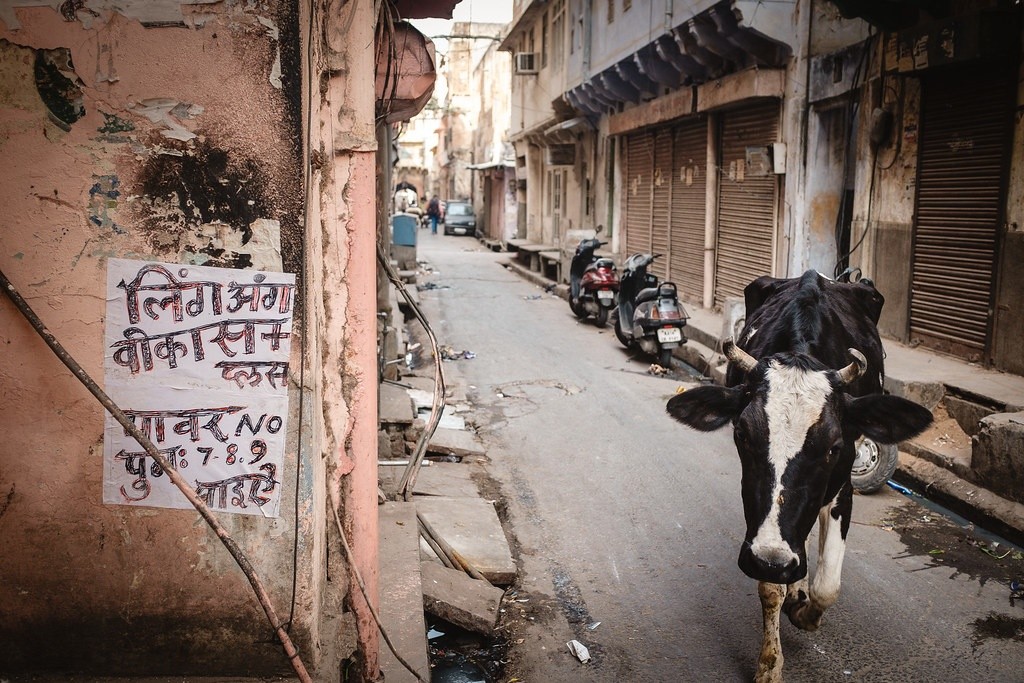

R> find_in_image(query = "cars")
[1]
[443,202,478,237]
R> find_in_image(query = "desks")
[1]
[508,239,561,283]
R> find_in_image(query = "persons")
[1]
[401,199,407,212]
[427,194,442,235]
[410,200,417,207]
[418,196,429,229]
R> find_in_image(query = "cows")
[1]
[665,269,937,683]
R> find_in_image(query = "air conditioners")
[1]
[513,52,539,75]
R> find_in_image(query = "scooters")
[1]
[610,252,693,369]
[565,225,623,328]
